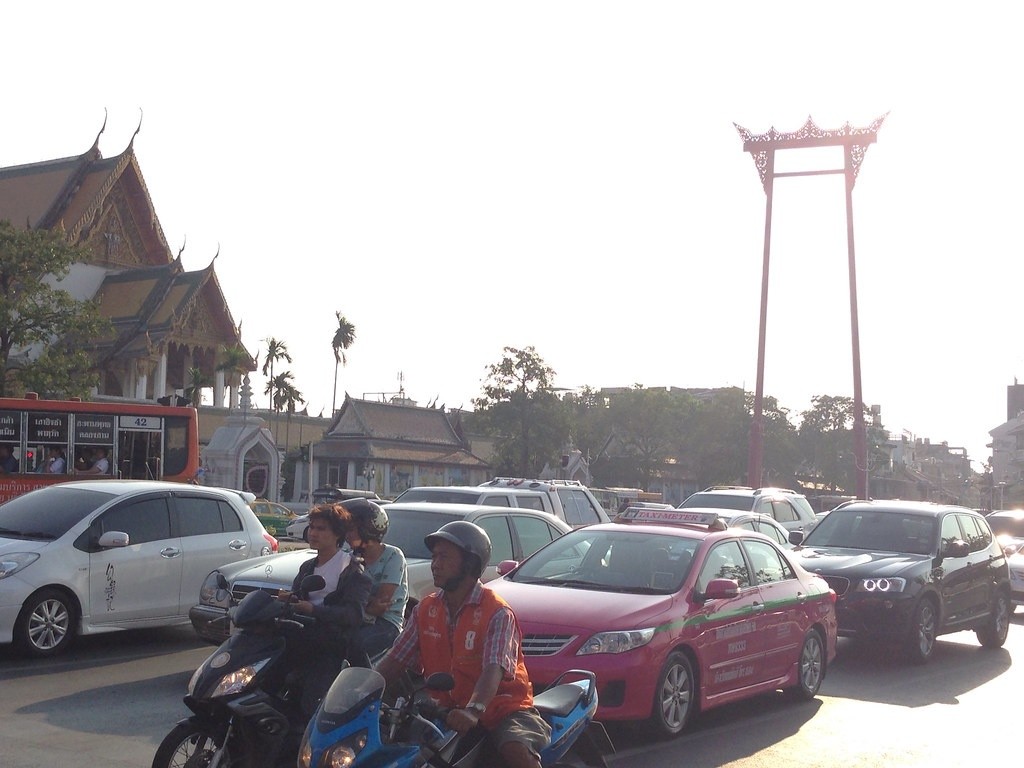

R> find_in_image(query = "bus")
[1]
[0,392,201,504]
[588,485,663,522]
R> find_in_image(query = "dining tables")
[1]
[749,551,769,570]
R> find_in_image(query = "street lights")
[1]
[998,482,1006,509]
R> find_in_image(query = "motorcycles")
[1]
[152,572,391,768]
[291,646,618,768]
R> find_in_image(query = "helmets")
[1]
[336,498,390,543]
[424,520,492,578]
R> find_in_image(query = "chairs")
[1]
[696,550,725,587]
[636,546,679,589]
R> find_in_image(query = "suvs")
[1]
[676,484,820,536]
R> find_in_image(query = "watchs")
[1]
[466,702,486,713]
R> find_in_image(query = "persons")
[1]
[75,447,109,474]
[278,505,373,730]
[0,442,19,474]
[35,445,66,473]
[189,456,206,485]
[356,521,552,768]
[617,498,631,513]
[345,499,409,665]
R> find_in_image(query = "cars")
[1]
[185,502,608,654]
[0,479,280,659]
[286,500,393,544]
[249,497,298,537]
[984,510,1024,613]
[391,476,612,531]
[790,498,1012,664]
[687,507,796,552]
[480,505,840,739]
